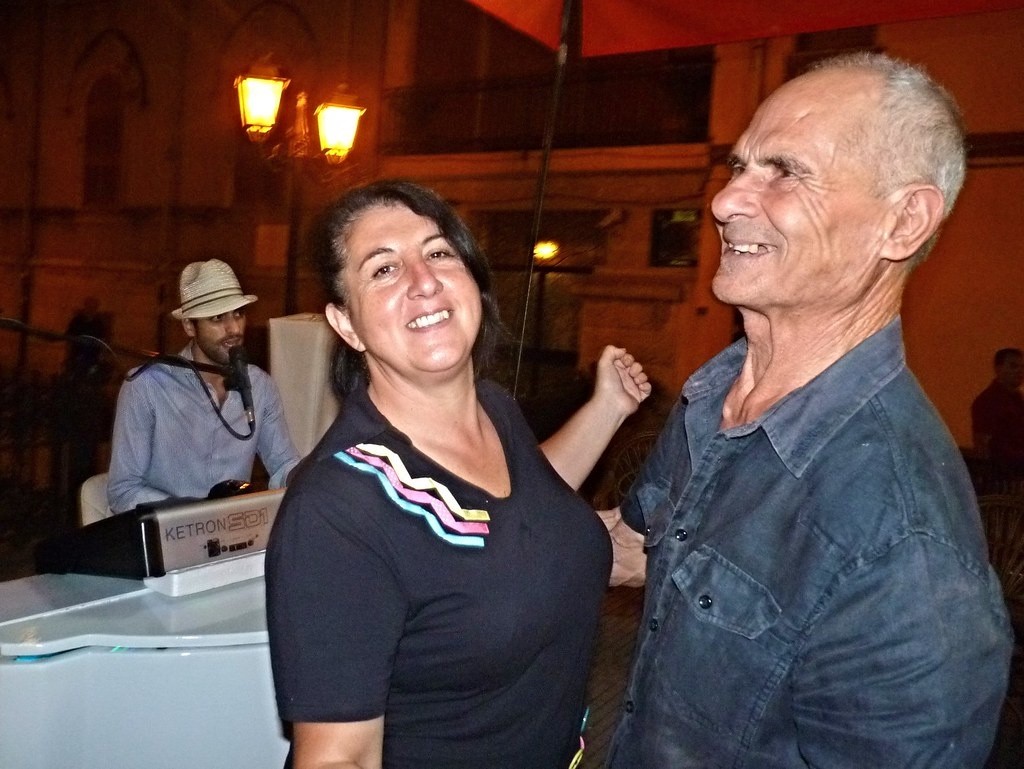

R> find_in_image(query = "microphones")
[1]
[228,344,257,433]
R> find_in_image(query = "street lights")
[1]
[232,60,369,312]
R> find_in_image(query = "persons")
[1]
[265,179,651,769]
[971,348,1024,496]
[107,258,302,516]
[596,52,1013,769]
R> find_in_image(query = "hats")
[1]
[170,257,258,319]
